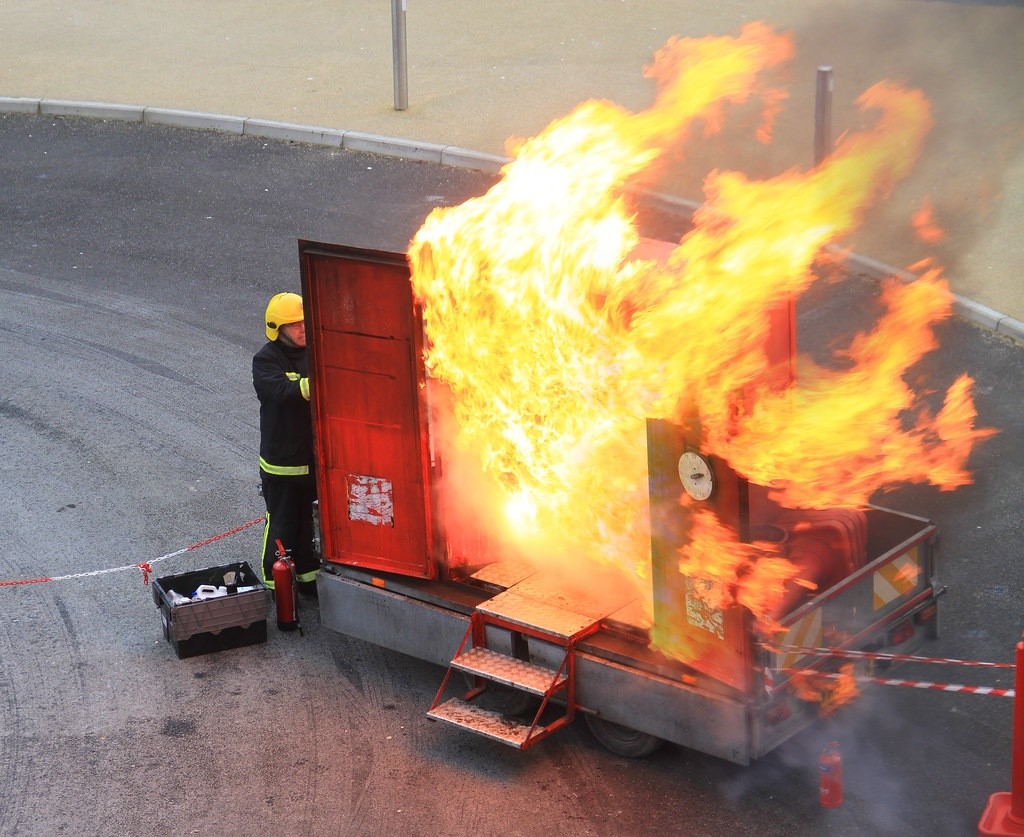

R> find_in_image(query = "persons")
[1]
[251,292,320,600]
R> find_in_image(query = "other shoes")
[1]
[295,578,319,600]
[272,585,301,609]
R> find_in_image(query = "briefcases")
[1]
[152,560,270,660]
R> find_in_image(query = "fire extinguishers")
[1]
[272,538,298,631]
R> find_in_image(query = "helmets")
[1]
[265,292,305,343]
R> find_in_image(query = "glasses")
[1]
[280,319,305,330]
